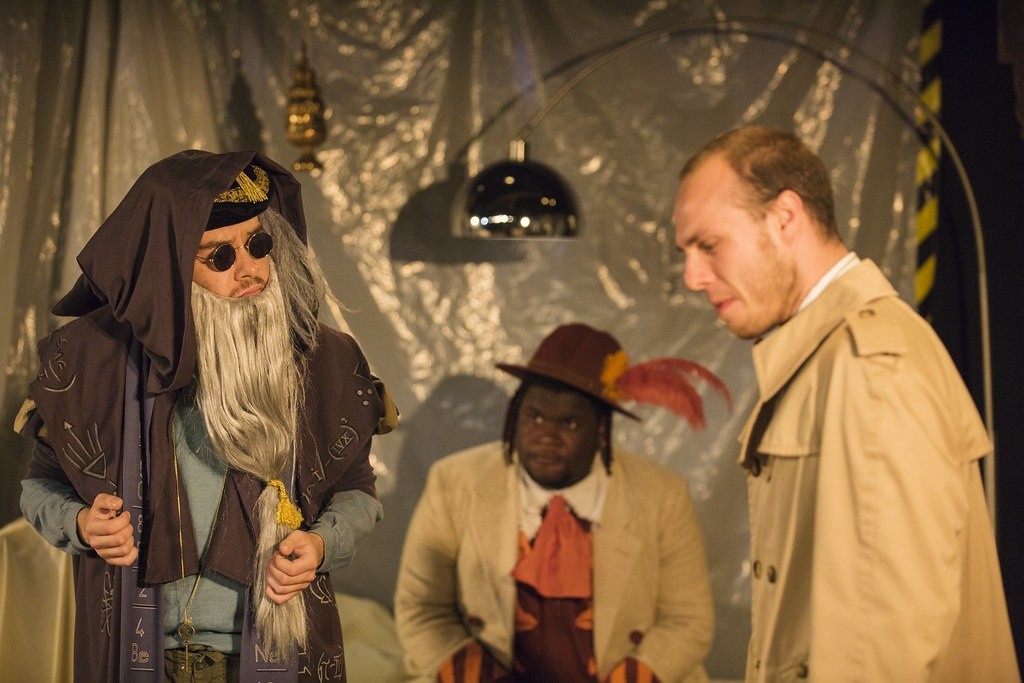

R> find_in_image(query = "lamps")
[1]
[451,16,995,532]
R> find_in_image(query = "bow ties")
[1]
[510,494,594,600]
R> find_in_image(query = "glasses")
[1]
[196,229,276,271]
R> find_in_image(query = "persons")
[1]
[672,126,1022,683]
[394,322,733,683]
[13,149,399,683]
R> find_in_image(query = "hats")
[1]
[493,321,646,425]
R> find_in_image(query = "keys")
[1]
[177,623,197,674]
